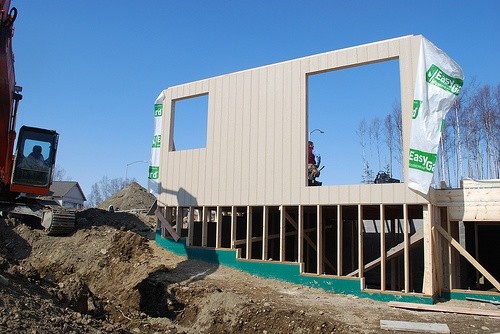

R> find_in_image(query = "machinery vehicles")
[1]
[0,0,76,235]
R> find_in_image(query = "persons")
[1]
[306,140,322,186]
[17,144,48,171]
